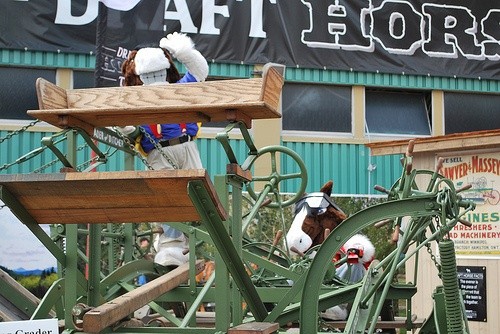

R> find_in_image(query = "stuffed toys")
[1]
[283,180,384,319]
[114,31,211,276]
